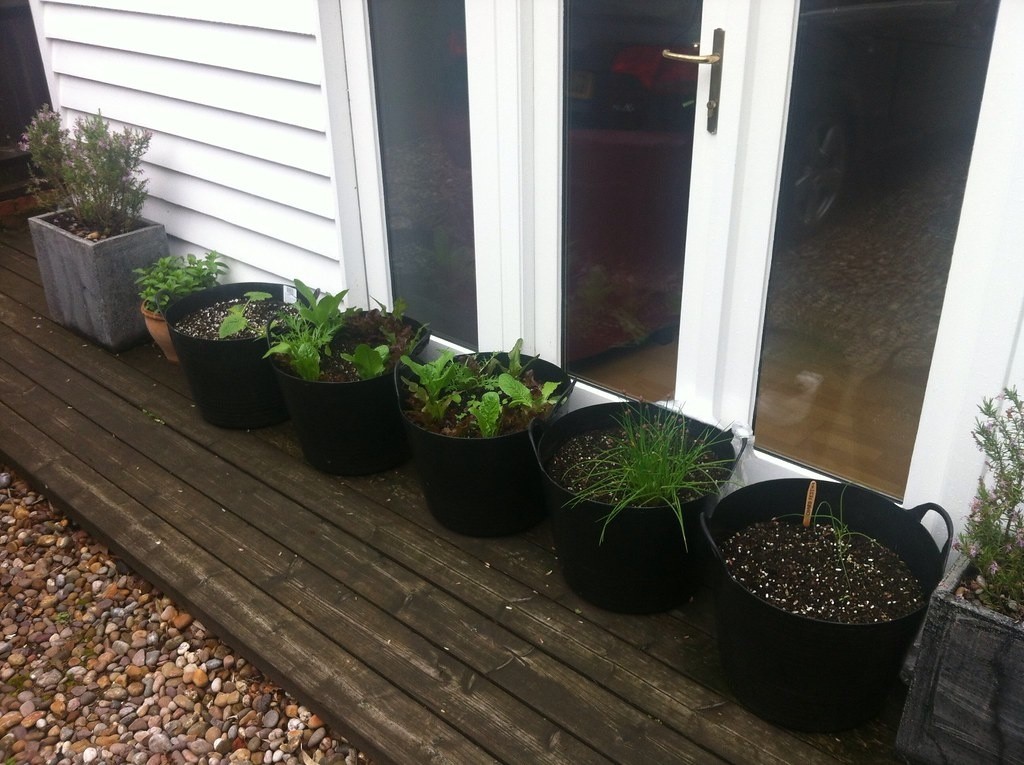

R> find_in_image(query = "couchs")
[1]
[423,113,692,361]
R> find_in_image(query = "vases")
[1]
[28,201,170,354]
[267,308,429,475]
[155,282,312,431]
[701,478,953,731]
[531,400,753,618]
[894,546,1023,765]
[394,352,579,538]
[141,299,181,361]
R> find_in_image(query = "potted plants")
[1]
[17,104,168,355]
[701,478,954,732]
[265,278,431,475]
[129,250,228,362]
[155,282,312,432]
[394,338,575,535]
[528,398,749,618]
[891,387,1024,765]
